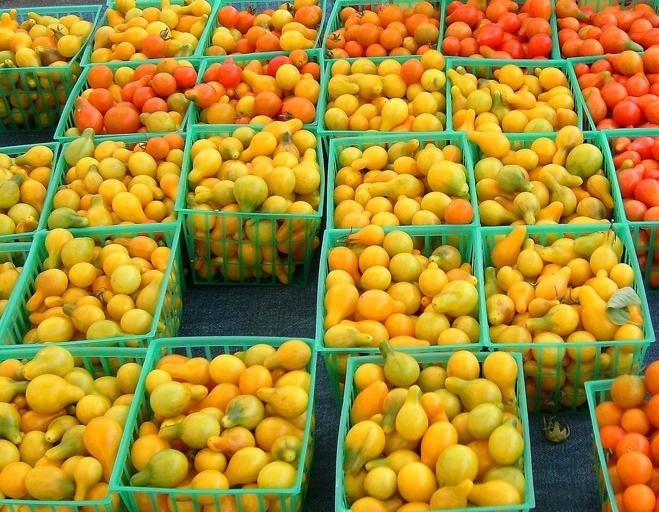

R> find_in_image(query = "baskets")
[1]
[0,0,659,510]
[0,0,659,512]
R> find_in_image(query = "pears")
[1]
[0,0,659,512]
